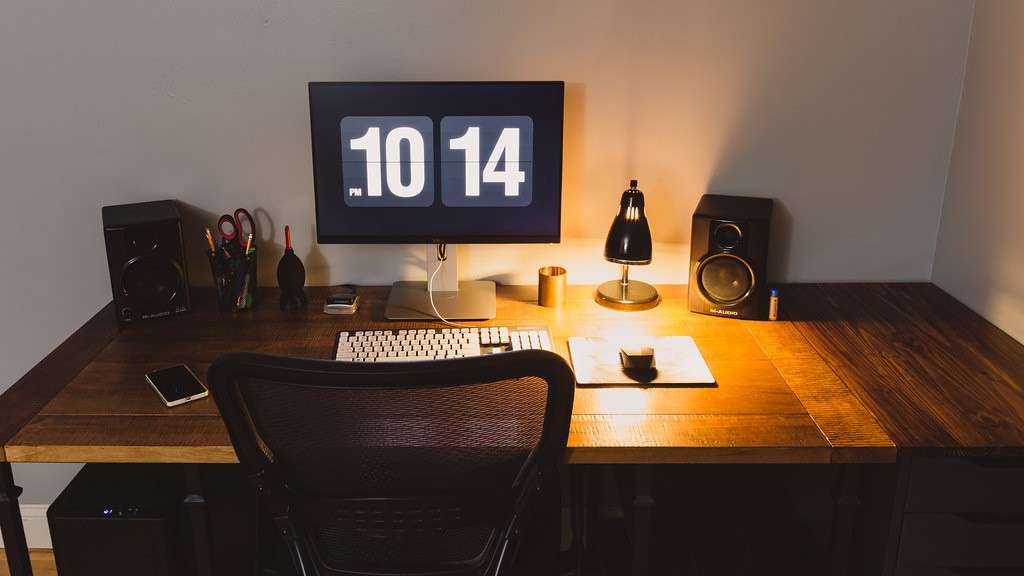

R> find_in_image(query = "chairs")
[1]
[204,347,577,573]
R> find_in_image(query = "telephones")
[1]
[145,363,209,407]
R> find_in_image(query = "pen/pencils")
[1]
[205,208,257,309]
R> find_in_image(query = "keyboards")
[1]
[332,326,557,363]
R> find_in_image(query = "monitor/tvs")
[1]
[307,80,565,244]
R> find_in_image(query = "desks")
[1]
[1,280,1024,575]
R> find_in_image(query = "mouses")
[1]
[619,345,656,369]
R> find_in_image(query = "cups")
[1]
[538,266,567,307]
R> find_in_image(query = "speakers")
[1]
[689,193,774,321]
[101,199,191,326]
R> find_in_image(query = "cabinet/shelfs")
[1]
[830,455,1023,574]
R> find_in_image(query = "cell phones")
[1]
[145,362,208,407]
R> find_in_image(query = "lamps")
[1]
[594,180,661,313]
